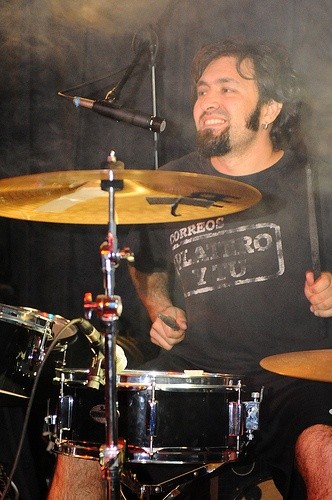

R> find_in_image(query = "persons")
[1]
[44,37,332,500]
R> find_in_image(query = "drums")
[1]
[45,365,265,465]
[0,303,79,400]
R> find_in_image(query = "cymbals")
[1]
[0,167,263,225]
[259,349,332,382]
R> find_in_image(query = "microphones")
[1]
[72,96,166,134]
[81,318,127,374]
